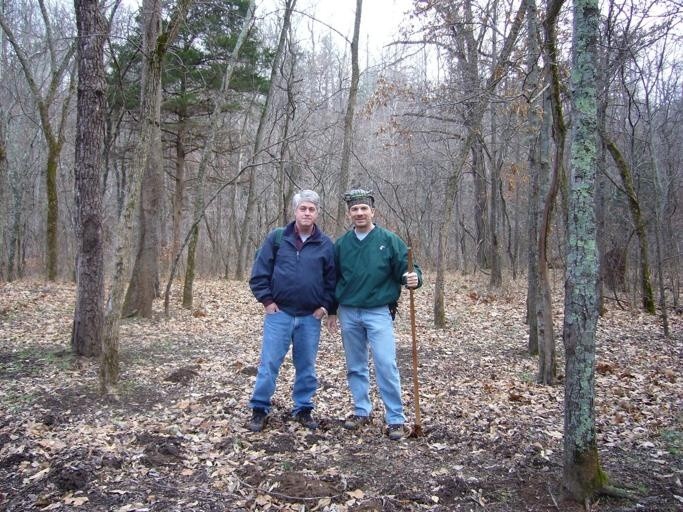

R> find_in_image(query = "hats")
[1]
[344,190,374,208]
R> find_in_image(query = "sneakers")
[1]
[294,411,318,429]
[388,424,404,439]
[344,416,370,429]
[250,411,264,431]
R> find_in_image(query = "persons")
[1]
[326,188,422,439]
[249,190,337,432]
